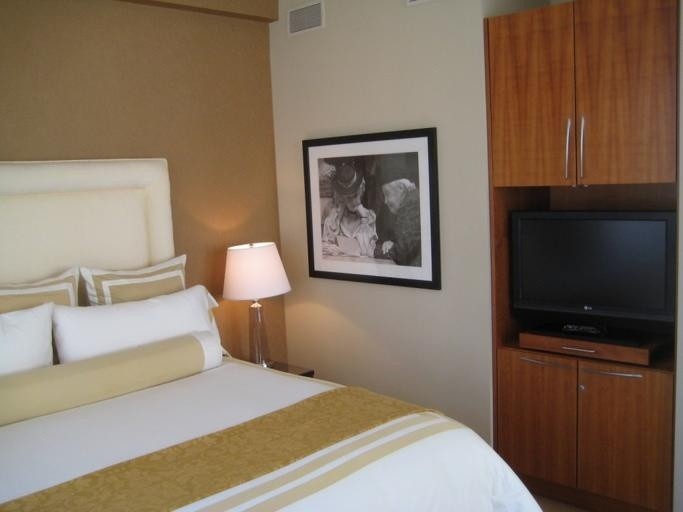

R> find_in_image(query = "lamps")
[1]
[222,242,291,368]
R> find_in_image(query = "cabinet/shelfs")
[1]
[484,0,678,189]
[484,350,678,512]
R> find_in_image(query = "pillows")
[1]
[0,255,224,427]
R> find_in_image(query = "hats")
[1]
[333,166,364,195]
[377,165,412,185]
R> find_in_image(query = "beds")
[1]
[0,156,542,512]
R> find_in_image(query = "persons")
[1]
[322,165,377,259]
[373,172,421,267]
[319,158,337,223]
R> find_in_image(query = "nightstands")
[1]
[273,361,315,378]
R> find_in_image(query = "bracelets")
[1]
[359,216,369,219]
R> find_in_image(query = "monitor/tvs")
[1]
[507,210,676,347]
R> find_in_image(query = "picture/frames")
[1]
[302,127,443,292]
